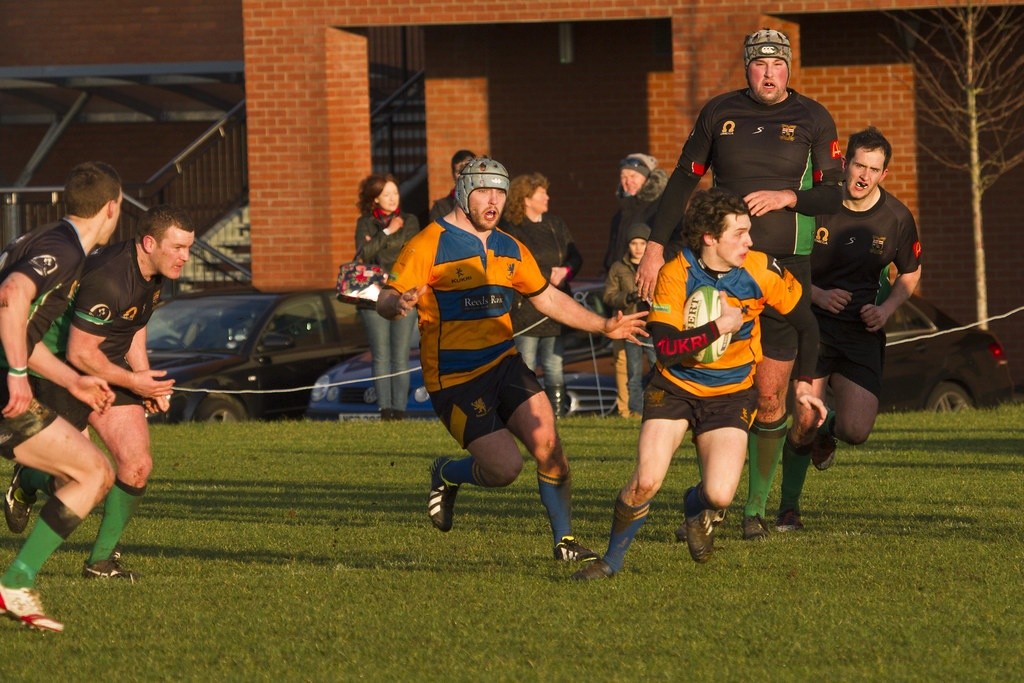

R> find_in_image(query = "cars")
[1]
[299,274,1012,420]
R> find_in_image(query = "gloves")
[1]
[637,302,650,320]
[627,290,640,304]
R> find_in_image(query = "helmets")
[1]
[455,157,510,214]
[746,29,791,82]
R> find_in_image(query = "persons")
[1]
[506,170,584,419]
[775,125,923,532]
[355,173,420,420]
[4,206,195,580]
[429,150,477,222]
[635,27,843,538]
[375,155,649,561]
[602,223,651,417]
[0,162,123,634]
[602,153,668,417]
[574,190,828,581]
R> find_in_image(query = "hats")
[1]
[627,223,651,244]
[618,153,658,178]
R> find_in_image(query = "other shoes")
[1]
[628,411,639,417]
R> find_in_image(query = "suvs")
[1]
[121,283,368,422]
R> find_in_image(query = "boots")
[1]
[543,382,563,419]
[379,409,405,418]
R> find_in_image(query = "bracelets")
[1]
[8,366,28,377]
[383,229,389,235]
[566,266,573,281]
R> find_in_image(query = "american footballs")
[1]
[683,284,733,365]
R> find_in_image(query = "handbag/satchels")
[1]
[335,261,388,306]
[560,283,578,335]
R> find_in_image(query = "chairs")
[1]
[213,310,328,358]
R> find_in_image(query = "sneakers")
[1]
[742,512,767,540]
[553,535,600,563]
[776,508,804,533]
[812,406,837,469]
[570,559,614,581]
[683,487,713,564]
[5,464,37,534]
[427,457,459,533]
[83,551,135,580]
[0,584,64,634]
[675,509,728,541]
